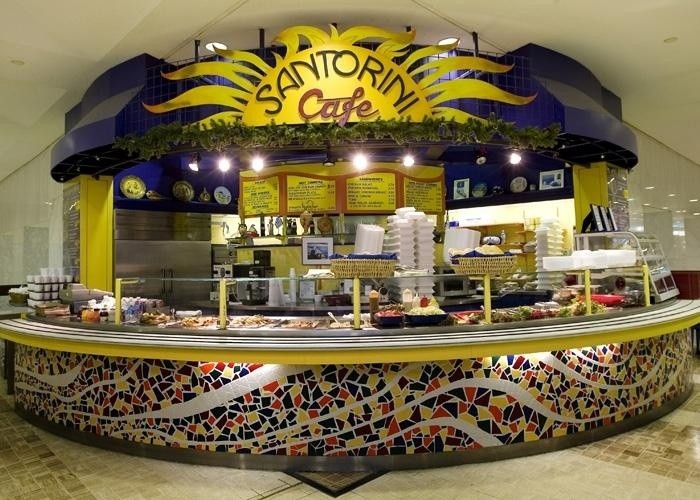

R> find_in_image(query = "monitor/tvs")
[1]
[253,251,271,266]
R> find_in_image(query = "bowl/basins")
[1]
[373,310,403,326]
[324,294,348,306]
[404,310,449,325]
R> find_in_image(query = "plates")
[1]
[471,183,487,198]
[213,185,232,205]
[172,180,196,201]
[120,174,147,200]
[510,176,528,193]
[577,293,624,307]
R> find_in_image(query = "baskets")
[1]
[449,255,518,279]
[331,258,400,280]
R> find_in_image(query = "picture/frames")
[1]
[582,202,618,231]
[300,236,334,266]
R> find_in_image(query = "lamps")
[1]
[474,146,486,165]
[323,146,334,166]
[189,151,200,173]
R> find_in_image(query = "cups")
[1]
[26,266,73,308]
[268,267,298,307]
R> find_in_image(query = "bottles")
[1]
[286,219,297,235]
[500,230,506,245]
[367,289,380,324]
[310,218,315,235]
[360,277,388,295]
[81,296,147,324]
